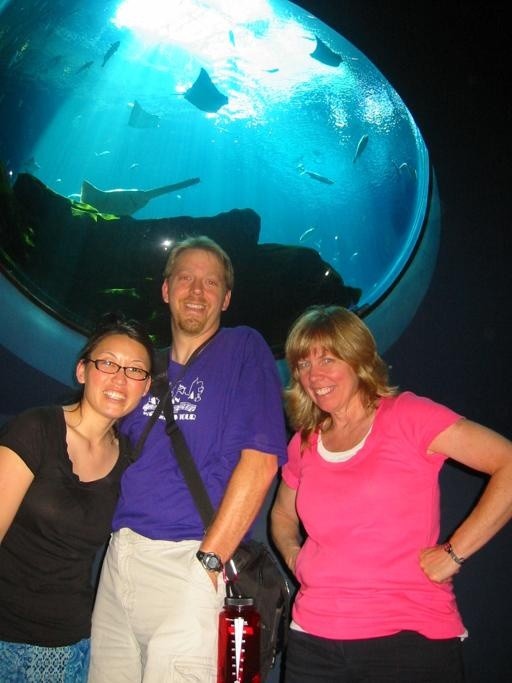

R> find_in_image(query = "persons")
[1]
[87,236,290,681]
[267,304,510,682]
[0,316,159,683]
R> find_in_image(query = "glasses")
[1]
[87,360,151,381]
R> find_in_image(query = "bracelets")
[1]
[443,542,466,564]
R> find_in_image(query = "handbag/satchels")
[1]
[226,540,292,679]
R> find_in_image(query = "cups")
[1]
[216,592,263,682]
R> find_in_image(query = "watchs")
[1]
[195,550,225,574]
